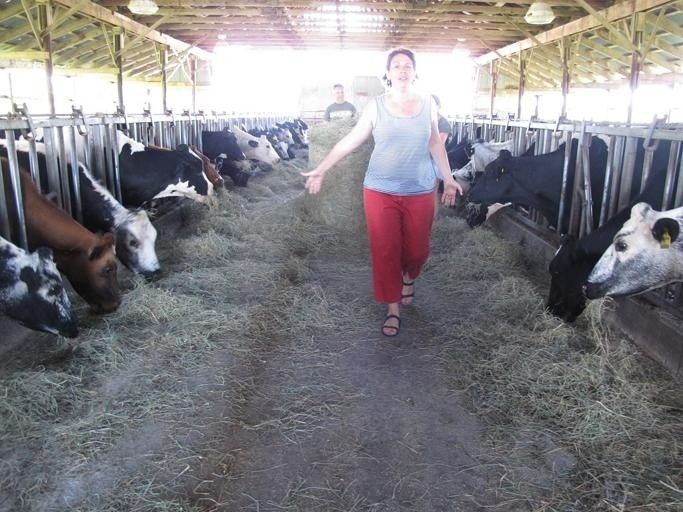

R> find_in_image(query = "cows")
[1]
[581,201,683,301]
[0,236,80,339]
[546,162,683,323]
[445,125,532,183]
[0,137,162,280]
[148,144,224,192]
[0,155,122,315]
[26,125,214,208]
[202,117,309,187]
[465,201,513,229]
[466,128,683,234]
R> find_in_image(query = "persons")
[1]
[323,84,356,124]
[430,94,451,160]
[297,47,465,337]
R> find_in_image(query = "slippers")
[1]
[381,315,400,337]
[399,282,414,307]
